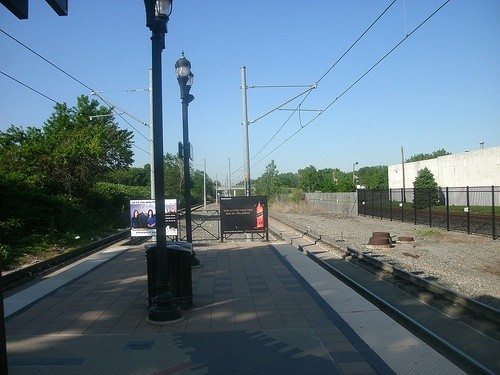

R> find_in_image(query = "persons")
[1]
[139,206,149,228]
[131,209,140,228]
[146,209,156,229]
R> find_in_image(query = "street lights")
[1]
[145,0,185,326]
[175,50,200,268]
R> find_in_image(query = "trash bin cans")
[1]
[144,240,194,309]
